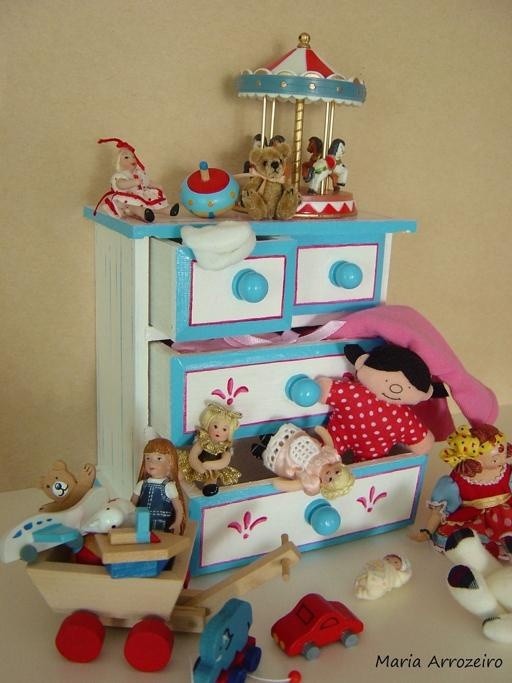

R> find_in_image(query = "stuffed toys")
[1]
[239,144,301,222]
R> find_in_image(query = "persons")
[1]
[313,343,450,467]
[0,402,414,682]
[235,34,369,218]
[92,134,181,223]
[440,529,511,643]
[406,422,511,563]
